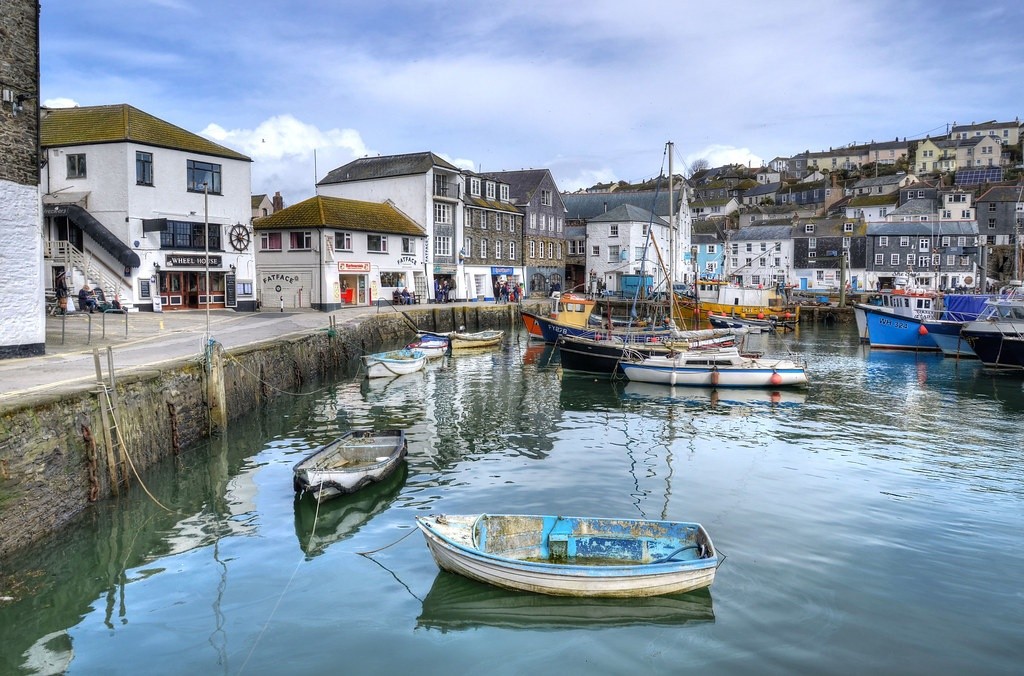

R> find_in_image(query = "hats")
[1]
[495,282,500,285]
[504,282,510,286]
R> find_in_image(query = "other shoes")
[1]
[90,310,94,313]
[400,303,404,305]
[495,302,500,304]
[504,301,507,304]
[407,303,410,305]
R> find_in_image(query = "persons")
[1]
[402,287,412,305]
[443,281,450,303]
[554,281,561,291]
[493,282,522,304]
[394,288,406,305]
[79,285,103,314]
[597,278,602,297]
[57,271,68,315]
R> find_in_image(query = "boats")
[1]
[414,512,727,599]
[404,337,450,359]
[360,347,428,379]
[293,428,410,504]
[518,138,1023,388]
[413,325,467,339]
[448,328,507,349]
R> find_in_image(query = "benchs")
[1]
[45,291,105,315]
[392,291,421,305]
